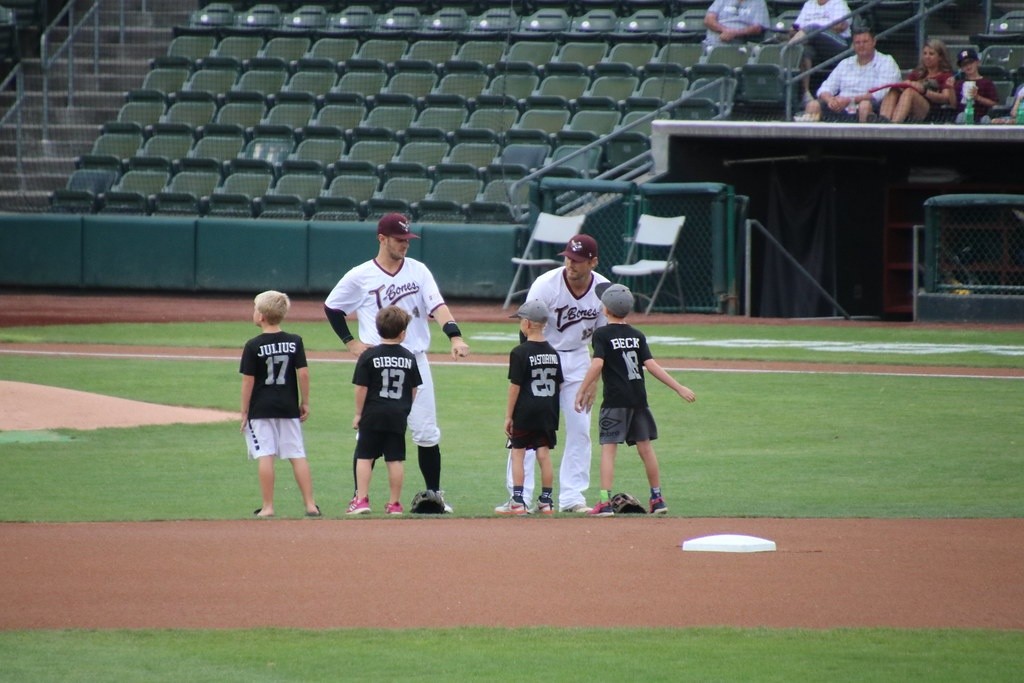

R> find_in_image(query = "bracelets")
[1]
[920,87,927,95]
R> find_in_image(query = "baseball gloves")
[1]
[410,489,445,514]
[611,492,648,514]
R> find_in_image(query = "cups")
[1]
[961,80,976,104]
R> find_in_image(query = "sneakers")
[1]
[385,502,404,515]
[649,496,668,514]
[585,500,614,517]
[528,498,555,515]
[345,494,371,515]
[495,497,536,516]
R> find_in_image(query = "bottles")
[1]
[1017,94,1024,124]
[964,97,977,125]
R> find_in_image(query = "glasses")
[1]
[960,59,976,68]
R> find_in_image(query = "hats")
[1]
[595,282,634,317]
[508,299,550,323]
[377,213,420,240]
[558,234,599,262]
[956,48,978,66]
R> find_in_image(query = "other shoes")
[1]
[565,504,593,513]
[800,91,814,108]
[436,490,453,514]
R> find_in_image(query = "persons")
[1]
[944,48,998,125]
[702,1,770,52]
[866,39,955,122]
[324,213,470,514]
[345,304,423,514]
[785,1,853,103]
[240,290,322,520]
[495,298,564,515]
[507,234,611,515]
[805,25,901,123]
[575,283,697,516]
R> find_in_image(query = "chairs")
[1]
[0,0,1024,316]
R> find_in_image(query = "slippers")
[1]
[253,508,262,515]
[304,505,322,517]
